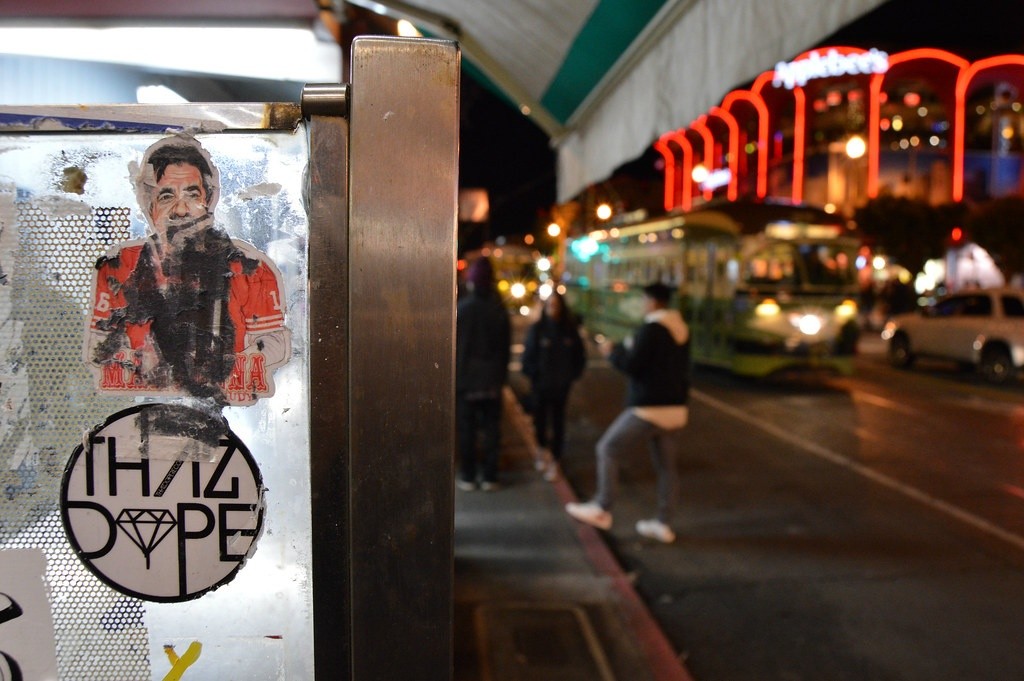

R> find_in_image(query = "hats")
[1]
[464,256,495,284]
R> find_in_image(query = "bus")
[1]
[462,246,543,315]
[558,199,862,378]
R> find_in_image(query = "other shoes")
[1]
[456,477,476,491]
[480,478,498,492]
[536,456,558,482]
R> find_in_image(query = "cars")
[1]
[879,290,1023,385]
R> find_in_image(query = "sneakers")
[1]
[635,519,672,542]
[567,501,613,530]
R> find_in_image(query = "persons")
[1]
[564,282,692,543]
[801,245,834,283]
[522,292,586,481]
[456,257,512,491]
[880,267,914,317]
